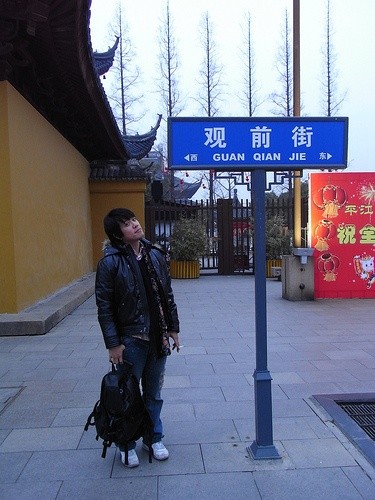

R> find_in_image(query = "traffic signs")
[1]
[166,117,349,170]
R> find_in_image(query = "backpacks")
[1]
[84,361,155,465]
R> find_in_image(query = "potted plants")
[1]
[249,215,292,278]
[166,216,214,279]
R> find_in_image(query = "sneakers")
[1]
[120,449,139,468]
[142,441,169,460]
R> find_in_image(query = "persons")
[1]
[94,207,180,467]
